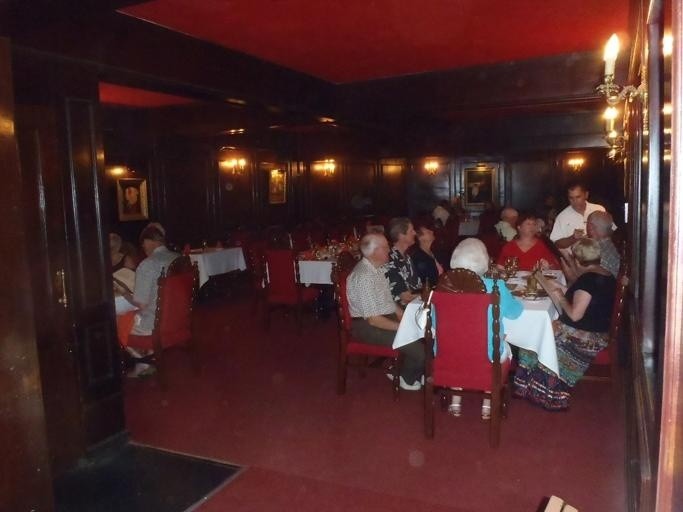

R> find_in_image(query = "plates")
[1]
[521,274,557,281]
[510,288,552,299]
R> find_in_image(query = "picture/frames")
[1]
[268,167,287,205]
[464,167,496,208]
[117,177,149,221]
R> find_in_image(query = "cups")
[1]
[525,277,537,291]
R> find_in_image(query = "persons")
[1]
[125,223,183,336]
[108,227,136,271]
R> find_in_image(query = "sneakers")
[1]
[126,361,159,379]
[386,365,422,392]
[447,403,462,417]
[480,406,491,420]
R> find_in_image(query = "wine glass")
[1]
[488,254,519,286]
[311,236,359,259]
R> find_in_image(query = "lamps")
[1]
[600,103,631,167]
[592,33,641,105]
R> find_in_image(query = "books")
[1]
[112,268,136,295]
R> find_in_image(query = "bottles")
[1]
[420,273,431,302]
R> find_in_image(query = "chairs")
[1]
[166,214,388,330]
[330,250,402,403]
[424,268,515,450]
[118,256,198,391]
[580,276,628,398]
[409,213,507,261]
